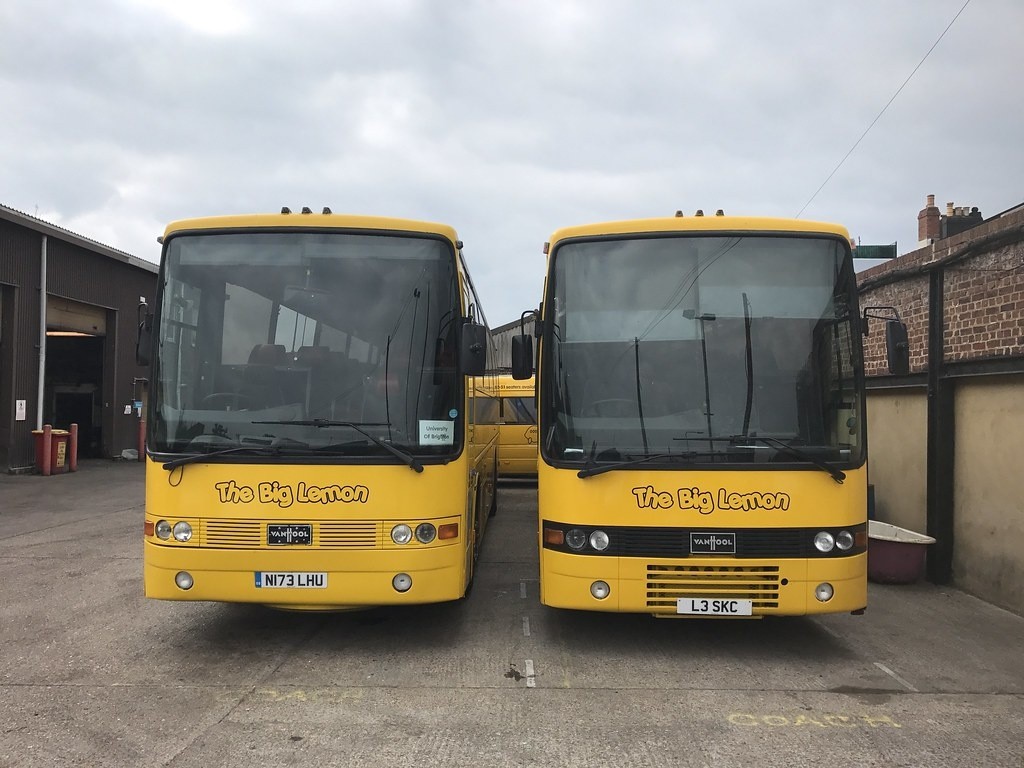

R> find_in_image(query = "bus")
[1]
[137,206,500,610]
[512,209,910,617]
[491,311,539,474]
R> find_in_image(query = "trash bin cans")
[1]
[32,429,71,475]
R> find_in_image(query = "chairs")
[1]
[216,344,376,419]
[580,376,644,420]
